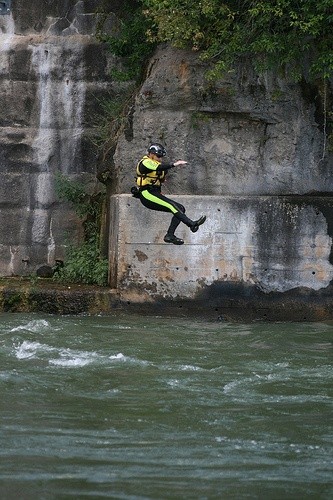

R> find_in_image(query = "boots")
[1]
[178,212,206,233]
[163,215,184,245]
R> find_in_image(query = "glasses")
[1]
[155,154,162,158]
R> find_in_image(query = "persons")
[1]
[136,143,206,245]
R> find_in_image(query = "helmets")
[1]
[147,143,167,156]
